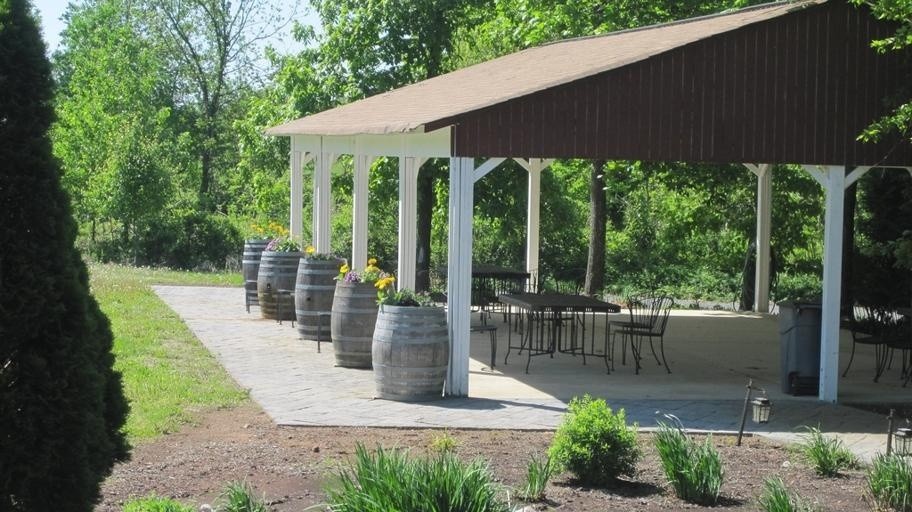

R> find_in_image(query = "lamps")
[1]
[885,408,912,458]
[736,377,773,446]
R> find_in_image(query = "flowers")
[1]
[246,223,291,239]
[331,257,392,286]
[303,245,339,262]
[374,275,433,311]
[265,236,303,253]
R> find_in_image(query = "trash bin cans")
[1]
[778,300,821,396]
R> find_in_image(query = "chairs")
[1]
[438,262,674,374]
[842,299,911,387]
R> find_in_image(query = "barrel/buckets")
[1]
[241,239,273,307]
[370,304,451,404]
[329,280,398,371]
[254,250,306,321]
[293,257,349,342]
